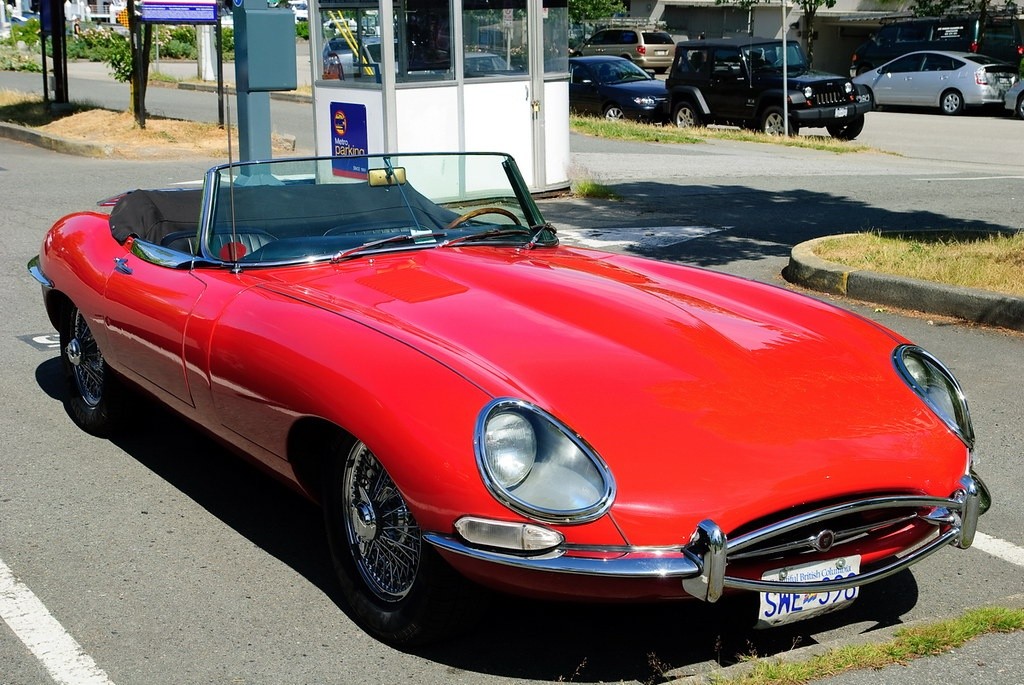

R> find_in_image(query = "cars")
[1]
[322,18,399,78]
[849,49,1021,117]
[439,52,516,76]
[544,54,669,126]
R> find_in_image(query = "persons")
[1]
[699,35,706,62]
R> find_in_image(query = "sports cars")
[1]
[26,148,989,651]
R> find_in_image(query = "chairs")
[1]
[323,219,432,236]
[747,48,766,69]
[690,51,706,70]
[163,226,279,258]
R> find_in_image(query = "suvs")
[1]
[666,34,873,139]
[845,11,1024,78]
[568,28,676,74]
